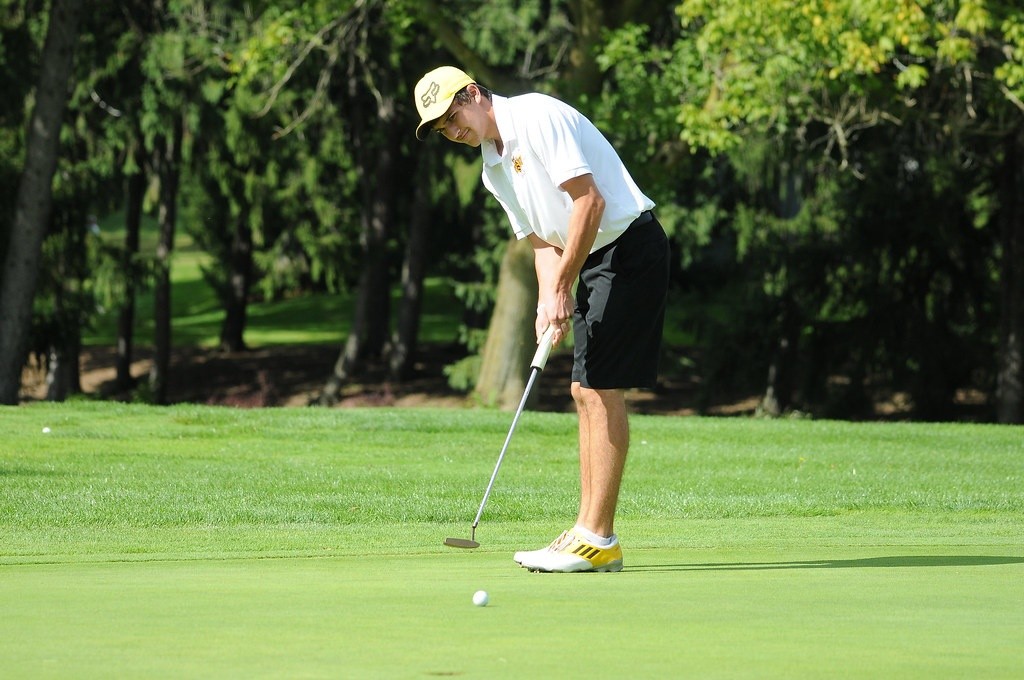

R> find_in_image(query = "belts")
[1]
[626,209,653,231]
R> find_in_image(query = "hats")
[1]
[413,65,477,140]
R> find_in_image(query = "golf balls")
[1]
[473,590,489,607]
[43,427,50,434]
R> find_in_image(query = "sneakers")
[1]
[513,528,623,574]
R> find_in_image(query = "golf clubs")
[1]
[444,325,555,548]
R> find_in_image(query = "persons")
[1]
[414,66,672,572]
